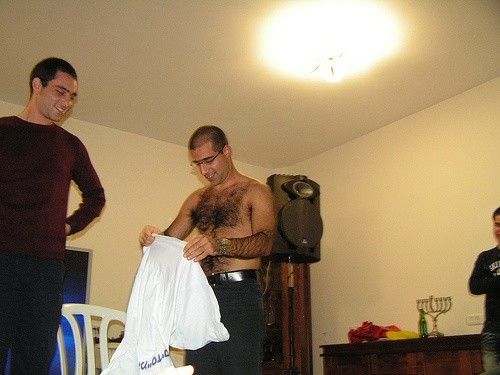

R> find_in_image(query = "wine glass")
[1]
[416,296,452,337]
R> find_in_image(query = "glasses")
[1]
[190,144,225,168]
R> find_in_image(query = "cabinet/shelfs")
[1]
[262,259,312,375]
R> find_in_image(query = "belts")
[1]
[205,270,257,287]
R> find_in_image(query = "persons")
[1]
[0,57,106,375]
[138,125,275,375]
[468,207,500,375]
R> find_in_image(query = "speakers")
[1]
[264,174,323,263]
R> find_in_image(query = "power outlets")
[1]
[466,313,484,325]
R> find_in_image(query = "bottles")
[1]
[418,309,428,338]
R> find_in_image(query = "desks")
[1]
[319,334,485,375]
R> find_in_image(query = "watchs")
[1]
[216,237,230,255]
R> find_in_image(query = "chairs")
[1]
[56,303,126,375]
